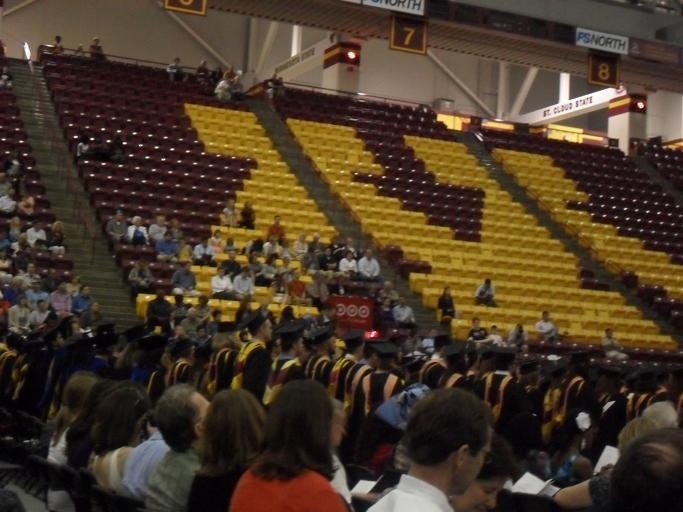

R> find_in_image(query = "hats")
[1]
[41,303,401,357]
[430,332,622,380]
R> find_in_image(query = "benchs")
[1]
[0,53,683,379]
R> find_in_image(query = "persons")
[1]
[0,315,366,510]
[275,75,285,97]
[107,199,420,336]
[438,287,456,318]
[341,312,681,510]
[76,134,125,163]
[52,34,65,53]
[1,159,104,343]
[73,42,85,55]
[266,73,277,98]
[88,36,104,59]
[165,56,187,82]
[193,60,243,102]
[475,278,500,308]
[0,67,14,89]
